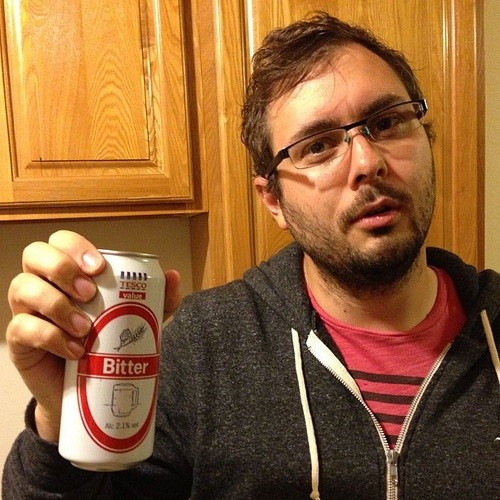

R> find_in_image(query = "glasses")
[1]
[266,97,429,177]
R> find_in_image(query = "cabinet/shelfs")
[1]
[1,0,488,293]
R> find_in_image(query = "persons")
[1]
[0,9,500,500]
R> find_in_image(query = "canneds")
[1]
[58,249,165,470]
